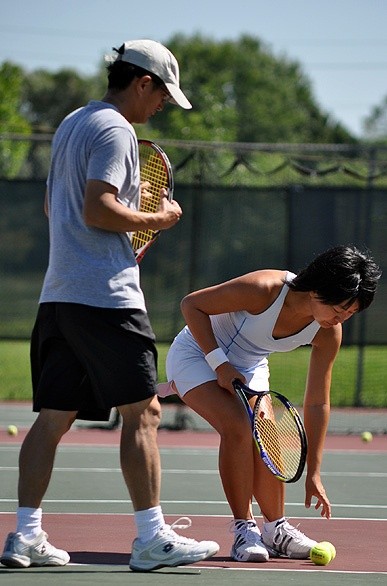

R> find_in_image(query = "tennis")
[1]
[7,425,17,438]
[361,432,373,442]
[310,541,336,565]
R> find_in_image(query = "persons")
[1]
[151,245,381,564]
[0,40,221,570]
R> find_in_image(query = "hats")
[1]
[104,40,193,110]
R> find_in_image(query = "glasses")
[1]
[158,83,173,104]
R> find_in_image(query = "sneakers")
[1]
[229,519,269,562]
[129,517,220,572]
[260,516,318,558]
[0,530,70,568]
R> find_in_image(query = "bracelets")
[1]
[203,346,228,370]
